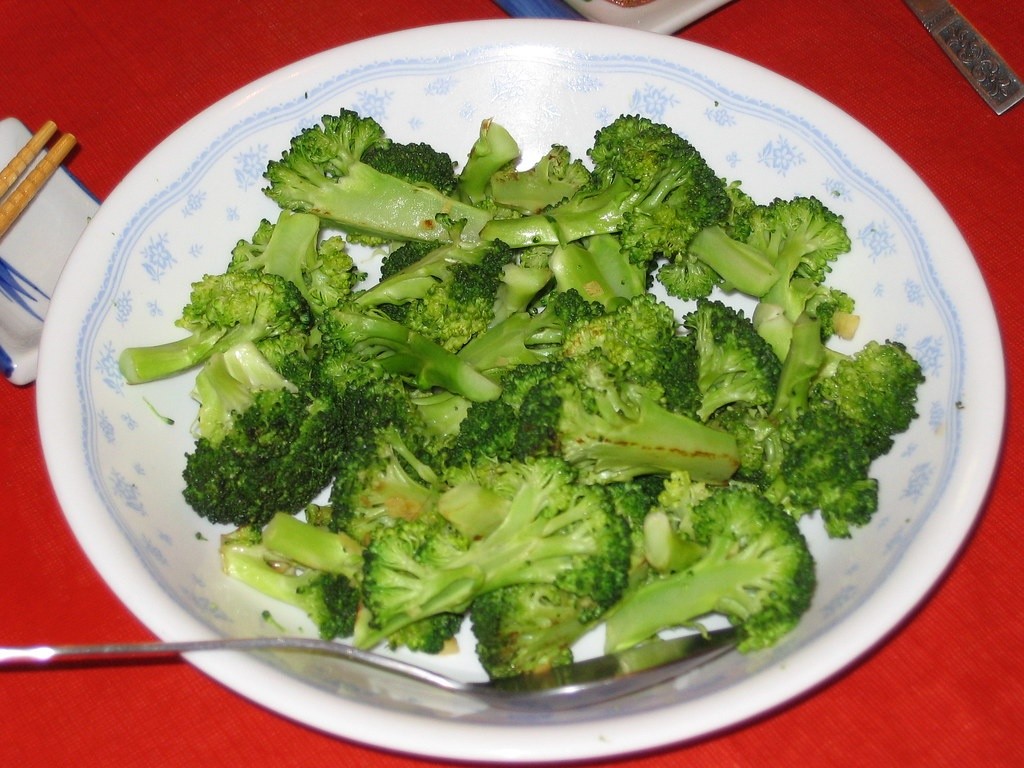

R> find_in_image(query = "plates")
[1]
[35,20,1007,763]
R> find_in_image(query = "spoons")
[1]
[0,628,746,709]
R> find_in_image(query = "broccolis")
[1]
[112,101,928,681]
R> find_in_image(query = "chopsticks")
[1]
[0,119,77,240]
